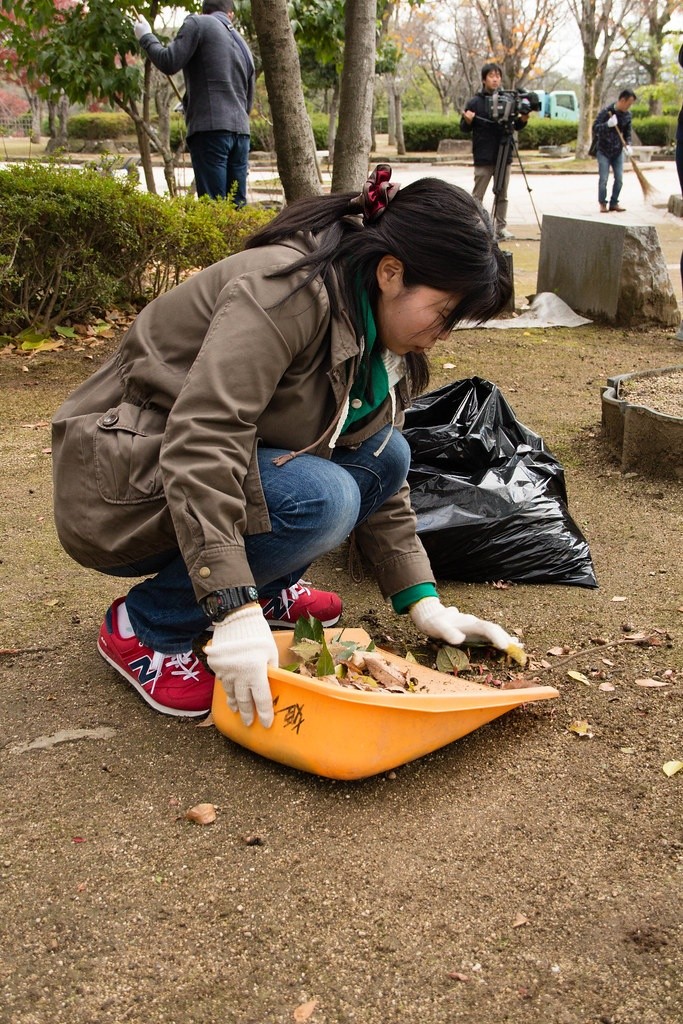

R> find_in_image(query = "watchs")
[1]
[199,587,257,623]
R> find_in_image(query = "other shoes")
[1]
[609,202,626,212]
[600,202,609,213]
[494,229,515,240]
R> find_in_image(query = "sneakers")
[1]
[206,578,344,629]
[96,595,218,717]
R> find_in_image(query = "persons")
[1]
[460,63,530,240]
[46,163,526,728]
[592,89,636,212]
[134,0,258,211]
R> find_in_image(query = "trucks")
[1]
[521,88,581,121]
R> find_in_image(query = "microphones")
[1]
[519,93,539,104]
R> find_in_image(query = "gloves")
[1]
[624,146,632,155]
[409,595,527,651]
[208,602,281,728]
[134,14,153,41]
[608,114,618,127]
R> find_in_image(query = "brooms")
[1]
[607,111,665,206]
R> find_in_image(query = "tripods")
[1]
[473,116,542,238]
